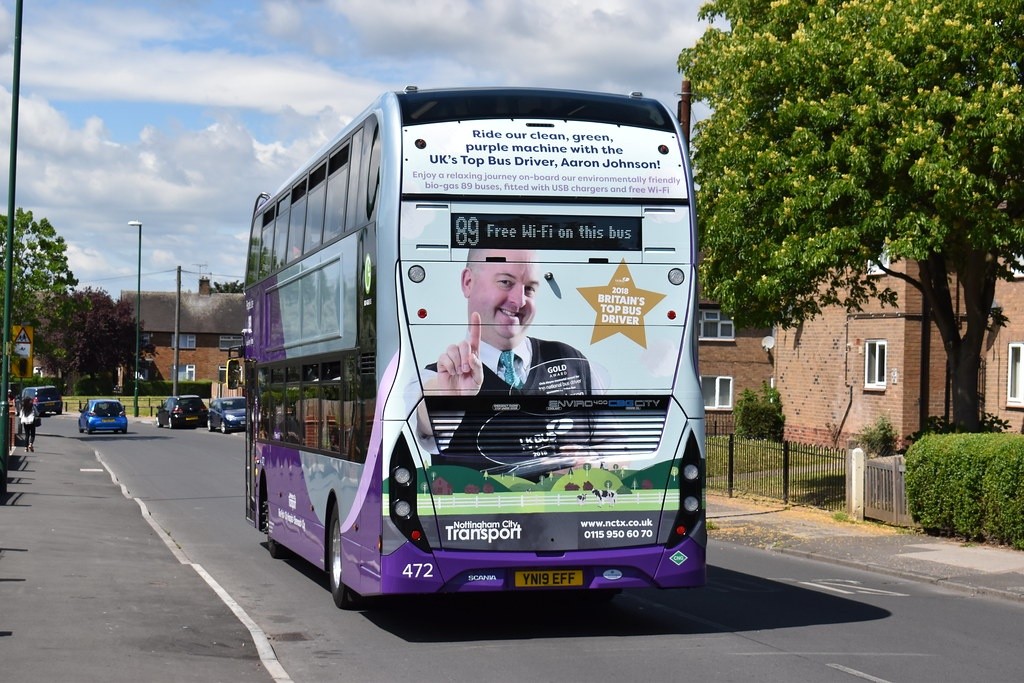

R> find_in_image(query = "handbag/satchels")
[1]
[35,415,41,427]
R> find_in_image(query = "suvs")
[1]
[14,386,63,416]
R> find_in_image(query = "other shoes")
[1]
[29,445,34,452]
[25,447,28,452]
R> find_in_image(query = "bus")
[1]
[220,80,707,609]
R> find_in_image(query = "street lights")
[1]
[128,221,142,416]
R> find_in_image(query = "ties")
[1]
[499,351,523,389]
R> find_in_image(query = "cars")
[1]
[207,397,249,434]
[78,400,127,434]
[155,396,209,429]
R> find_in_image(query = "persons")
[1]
[415,249,628,477]
[19,396,40,452]
